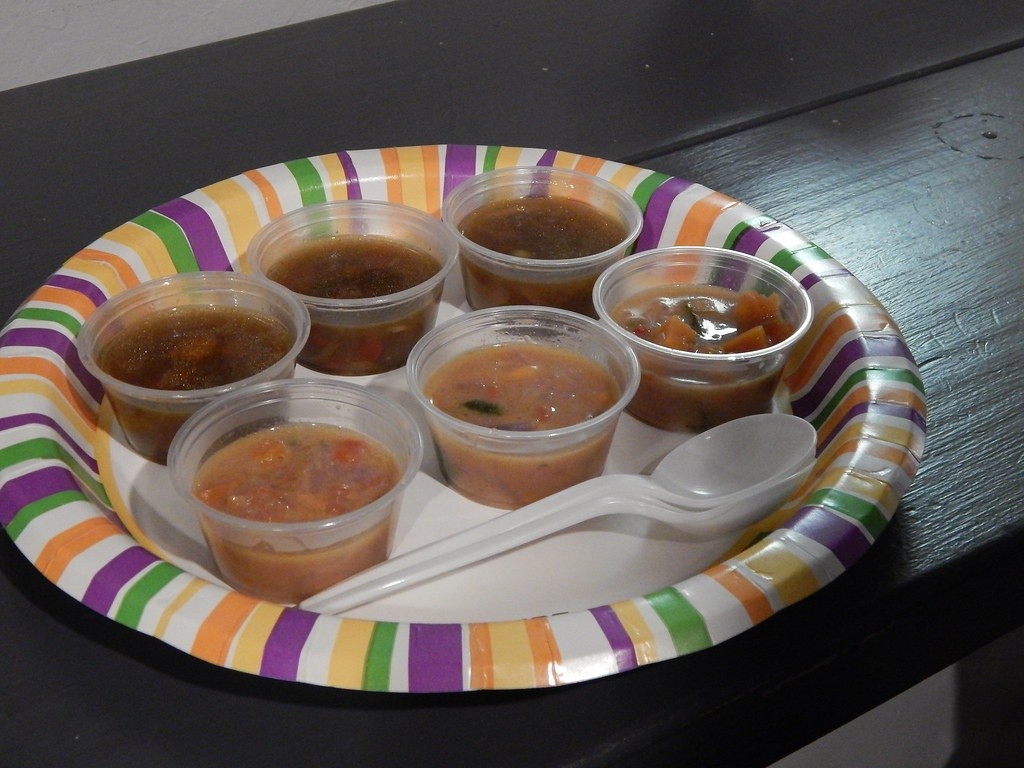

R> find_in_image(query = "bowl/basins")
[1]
[444,165,644,323]
[407,305,640,507]
[257,199,458,375]
[78,271,308,467]
[595,248,813,434]
[166,379,423,603]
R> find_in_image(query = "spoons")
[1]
[292,413,819,625]
